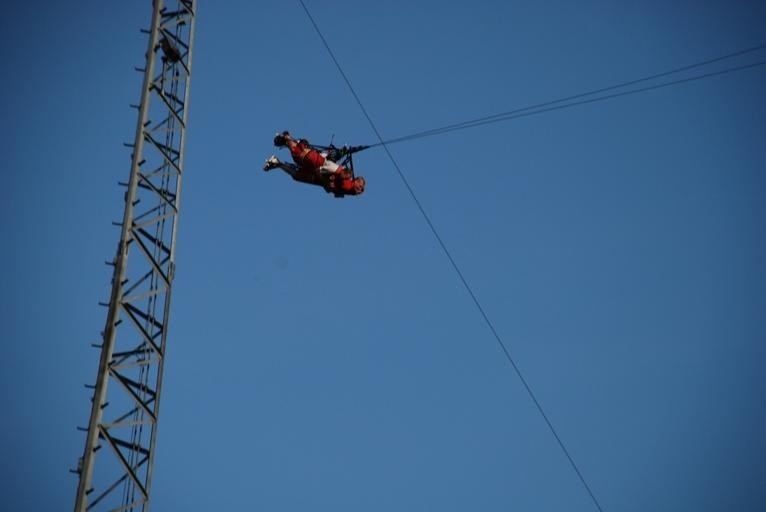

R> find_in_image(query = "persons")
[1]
[281,129,352,180]
[263,154,366,197]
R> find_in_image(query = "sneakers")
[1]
[274,131,289,146]
[265,156,279,170]
